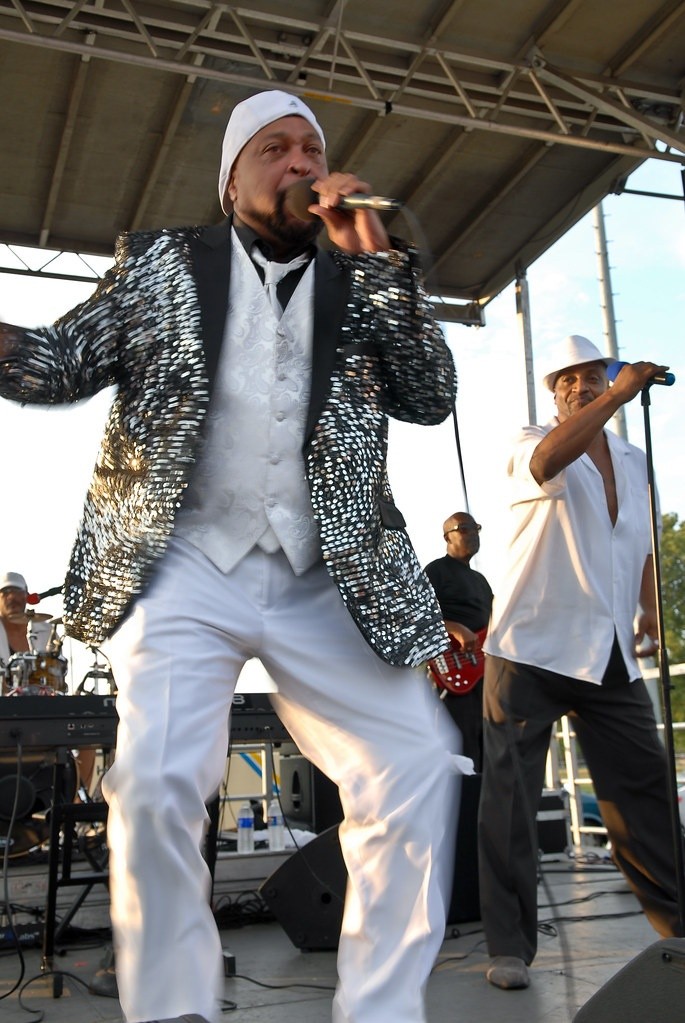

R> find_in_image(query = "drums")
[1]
[4,650,67,696]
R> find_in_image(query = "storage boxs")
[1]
[534,789,576,865]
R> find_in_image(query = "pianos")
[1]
[0,686,309,1004]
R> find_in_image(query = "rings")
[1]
[653,639,659,645]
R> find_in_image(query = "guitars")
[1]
[427,631,487,690]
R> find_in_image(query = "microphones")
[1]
[27,586,65,606]
[286,178,404,222]
[606,361,675,387]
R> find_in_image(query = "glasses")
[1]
[447,522,482,535]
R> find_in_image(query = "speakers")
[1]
[259,822,349,953]
[279,758,345,834]
[572,936,685,1023]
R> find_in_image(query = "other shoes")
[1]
[486,957,531,988]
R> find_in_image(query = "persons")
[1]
[0,90,460,1023]
[0,573,95,852]
[422,512,494,739]
[480,334,685,989]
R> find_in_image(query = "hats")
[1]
[217,88,325,213]
[0,572,28,592]
[545,335,617,391]
